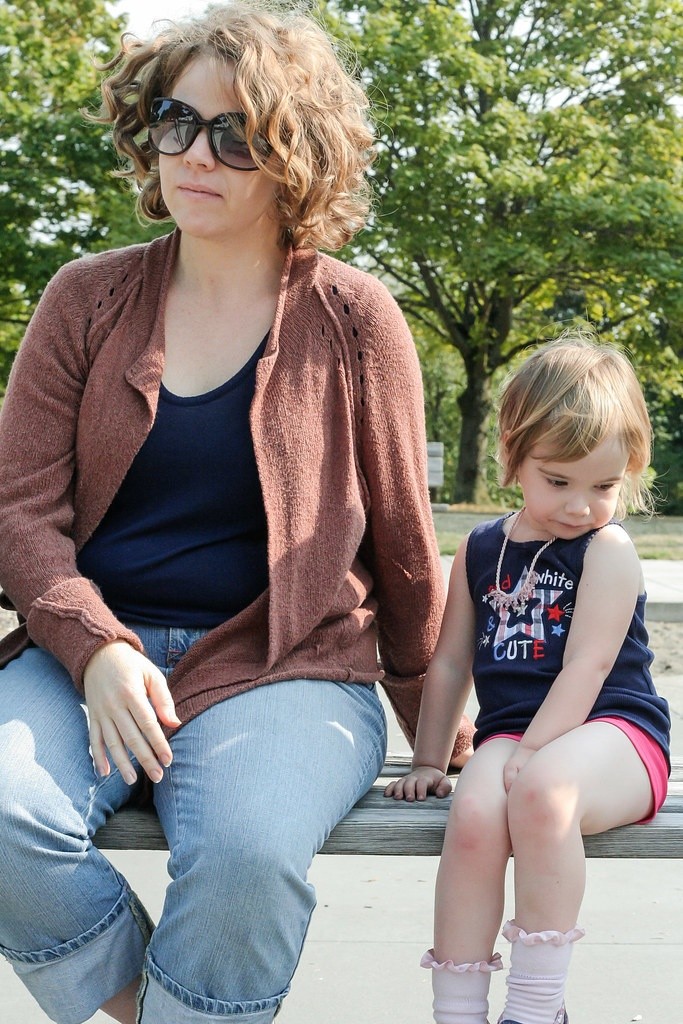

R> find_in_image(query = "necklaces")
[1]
[492,507,556,612]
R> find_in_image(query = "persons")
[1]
[0,4,477,1024]
[383,337,670,1024]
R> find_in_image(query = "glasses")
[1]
[148,96,276,171]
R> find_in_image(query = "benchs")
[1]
[87,755,683,860]
